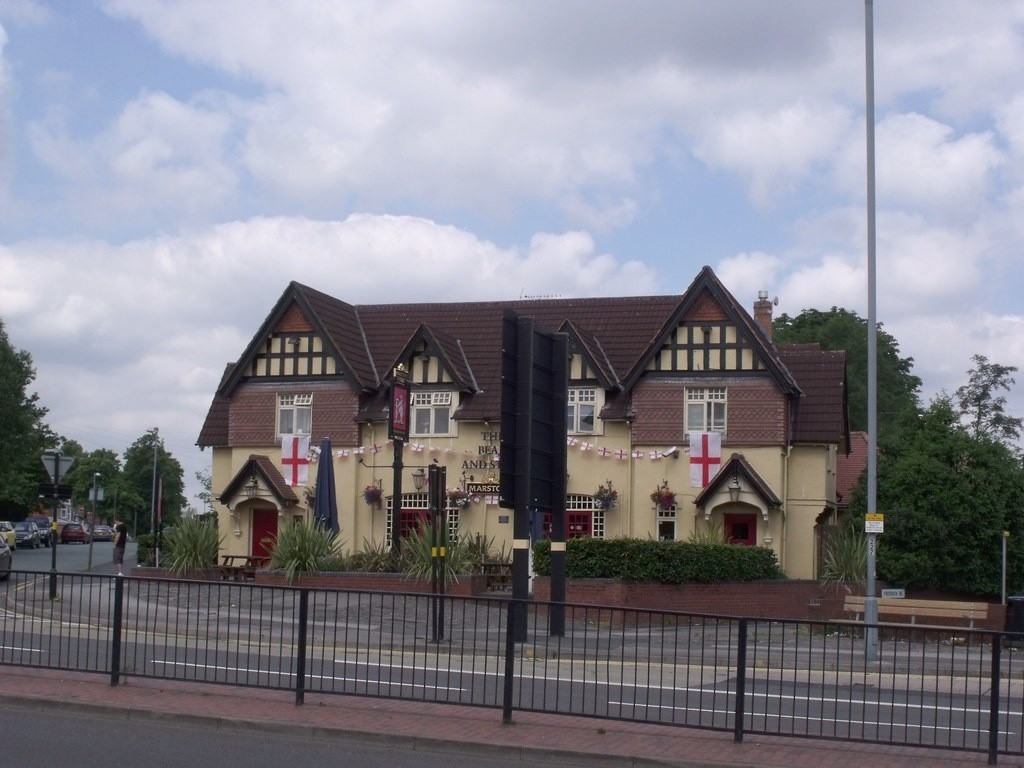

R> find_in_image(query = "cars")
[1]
[61,523,90,544]
[93,525,114,542]
[0,534,13,579]
[0,521,17,550]
[11,521,43,548]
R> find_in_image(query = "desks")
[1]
[481,562,515,589]
[221,555,272,565]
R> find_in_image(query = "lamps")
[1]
[417,355,431,360]
[288,336,302,345]
[701,325,713,331]
[243,475,258,500]
[728,476,740,504]
[414,467,426,489]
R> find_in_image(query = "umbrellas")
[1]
[313,437,341,535]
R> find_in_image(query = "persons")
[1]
[112,517,127,578]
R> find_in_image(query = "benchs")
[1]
[488,574,513,590]
[213,564,265,581]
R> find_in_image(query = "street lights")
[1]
[88,473,102,570]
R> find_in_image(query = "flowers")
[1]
[364,476,386,509]
[592,479,619,511]
[650,479,676,509]
[446,486,471,512]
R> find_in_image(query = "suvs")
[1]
[25,516,53,548]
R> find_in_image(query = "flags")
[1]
[689,432,721,488]
[309,435,679,506]
[281,436,309,487]
